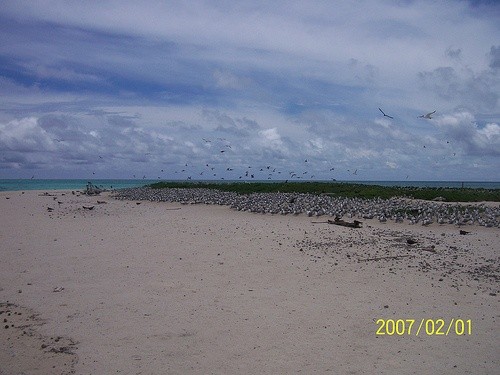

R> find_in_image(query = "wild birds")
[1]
[7,137,456,179]
[415,110,436,119]
[378,107,393,119]
[47,180,500,244]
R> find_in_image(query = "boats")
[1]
[328,219,363,228]
